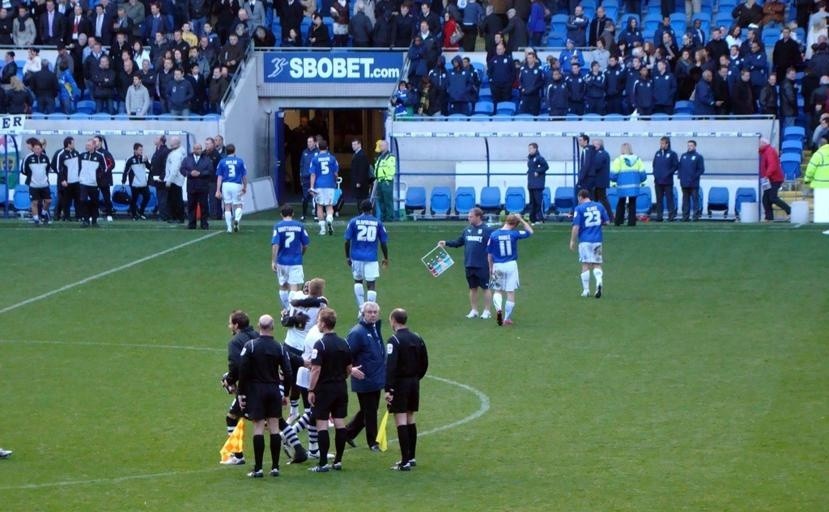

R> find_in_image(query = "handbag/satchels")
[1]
[450,26,465,44]
[113,186,131,205]
[23,102,31,113]
[333,22,349,35]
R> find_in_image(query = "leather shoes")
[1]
[372,445,382,452]
[347,440,356,447]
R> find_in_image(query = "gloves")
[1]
[291,300,299,307]
[294,312,310,324]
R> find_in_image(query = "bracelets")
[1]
[346,257,351,261]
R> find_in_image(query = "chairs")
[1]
[405,186,426,215]
[31,96,220,121]
[14,185,30,209]
[0,61,5,77]
[734,186,756,213]
[544,1,805,46]
[691,187,703,215]
[111,183,133,211]
[777,72,807,188]
[504,186,526,213]
[707,186,729,217]
[663,187,678,213]
[269,2,350,46]
[137,187,159,212]
[636,186,651,213]
[606,187,618,210]
[543,186,552,212]
[0,185,7,202]
[475,186,501,211]
[454,186,476,214]
[48,184,60,212]
[429,187,452,215]
[12,59,28,82]
[555,187,575,214]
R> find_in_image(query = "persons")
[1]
[269,203,310,310]
[760,114,829,235]
[526,134,705,226]
[22,136,247,232]
[427,250,445,270]
[437,206,497,320]
[484,210,535,326]
[568,189,612,299]
[220,277,428,477]
[344,200,389,319]
[392,1,828,128]
[300,135,396,235]
[0,0,272,125]
[250,0,397,48]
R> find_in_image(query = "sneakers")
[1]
[247,469,263,477]
[234,219,238,233]
[0,448,13,456]
[184,225,195,229]
[167,219,183,223]
[396,458,416,466]
[93,223,100,227]
[503,319,512,326]
[497,309,503,326]
[132,216,138,221]
[595,282,601,298]
[581,290,590,298]
[270,468,279,475]
[320,230,326,235]
[308,450,335,459]
[220,457,245,465]
[140,215,146,220]
[391,463,411,471]
[311,464,328,472]
[80,221,89,226]
[480,311,492,319]
[41,210,50,221]
[328,221,333,235]
[465,310,479,319]
[286,414,300,425]
[32,217,39,225]
[227,229,233,233]
[328,462,342,469]
[201,226,208,228]
[107,216,113,222]
[279,432,293,459]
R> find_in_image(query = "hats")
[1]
[295,313,304,328]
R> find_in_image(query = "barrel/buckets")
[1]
[740,201,757,223]
[791,200,810,226]
